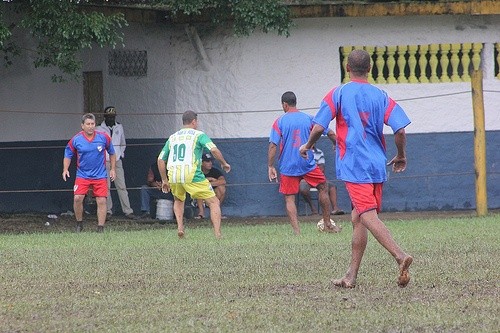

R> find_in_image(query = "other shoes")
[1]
[140,212,151,220]
[330,209,344,215]
[96,226,104,234]
[311,206,318,216]
[124,212,139,220]
[76,221,83,232]
[106,213,113,221]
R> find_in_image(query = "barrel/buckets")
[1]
[156,199,174,221]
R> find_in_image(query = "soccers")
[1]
[317,218,336,232]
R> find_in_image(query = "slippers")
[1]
[193,215,206,219]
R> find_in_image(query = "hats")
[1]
[202,153,213,160]
[104,106,117,116]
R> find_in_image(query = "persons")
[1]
[267,90,342,235]
[62,111,116,233]
[300,139,345,215]
[94,105,135,220]
[191,152,228,219]
[138,158,176,219]
[157,109,232,238]
[299,48,412,288]
